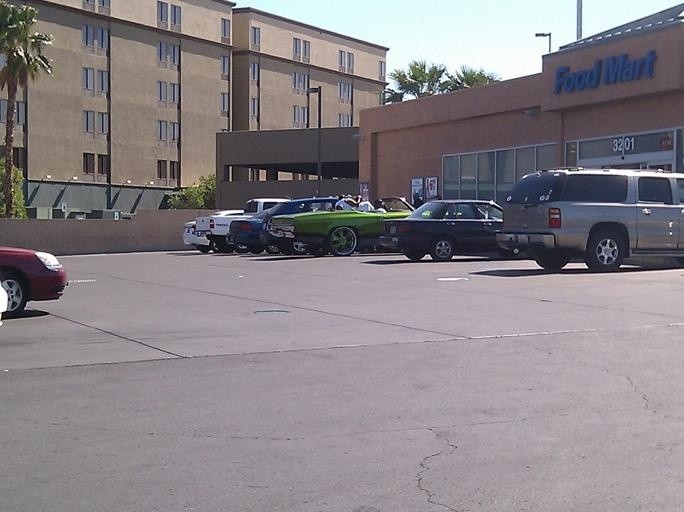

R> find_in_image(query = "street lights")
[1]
[536,33,552,53]
[307,86,322,196]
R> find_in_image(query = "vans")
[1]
[495,166,684,272]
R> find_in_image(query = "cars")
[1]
[183,197,503,261]
[0,246,68,314]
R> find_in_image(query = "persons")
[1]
[335,193,388,213]
[411,193,424,209]
